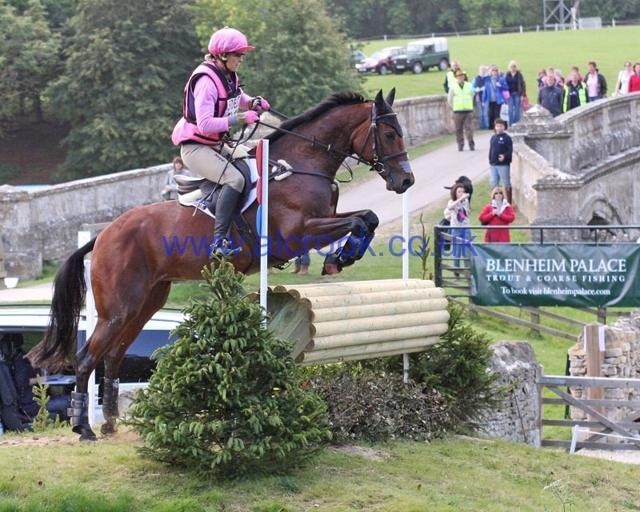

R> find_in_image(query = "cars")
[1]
[354,46,407,75]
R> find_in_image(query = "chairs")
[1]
[0,334,70,432]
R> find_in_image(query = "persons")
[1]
[489,119,512,205]
[443,59,640,151]
[171,25,270,260]
[444,183,471,281]
[166,157,192,201]
[479,187,515,242]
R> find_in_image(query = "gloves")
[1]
[256,97,271,114]
[245,110,259,124]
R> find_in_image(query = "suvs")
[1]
[391,37,451,74]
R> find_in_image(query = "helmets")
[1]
[207,26,255,56]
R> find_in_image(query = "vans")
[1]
[0,306,215,435]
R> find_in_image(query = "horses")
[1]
[23,86,415,442]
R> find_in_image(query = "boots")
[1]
[208,183,242,260]
